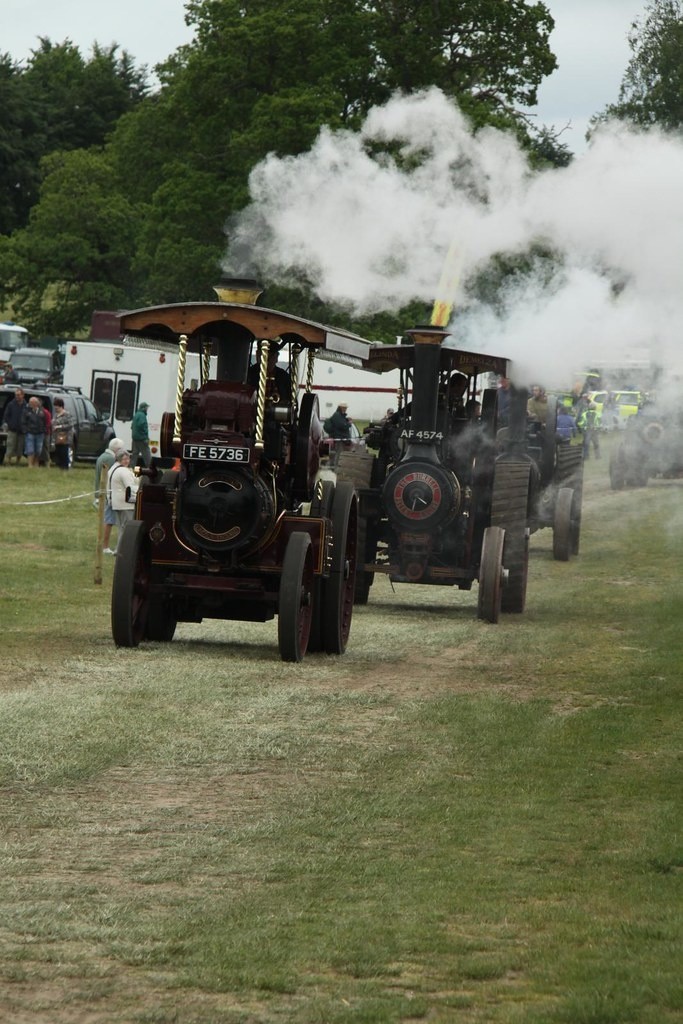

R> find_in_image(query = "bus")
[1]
[0,321,28,370]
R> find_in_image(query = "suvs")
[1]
[4,345,65,386]
[0,384,117,466]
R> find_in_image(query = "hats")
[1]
[339,401,348,408]
[140,402,150,408]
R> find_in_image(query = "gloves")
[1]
[144,439,149,446]
[2,423,8,431]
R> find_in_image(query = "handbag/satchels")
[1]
[349,422,362,445]
[125,486,140,503]
[56,424,67,445]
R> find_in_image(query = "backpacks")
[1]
[324,415,336,434]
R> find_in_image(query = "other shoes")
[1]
[103,548,113,554]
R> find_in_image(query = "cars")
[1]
[583,387,644,421]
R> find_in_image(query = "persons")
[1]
[0,360,19,385]
[129,402,152,468]
[94,437,140,555]
[496,364,658,462]
[381,408,395,419]
[2,386,74,471]
[440,372,482,436]
[321,402,361,439]
[245,337,299,416]
